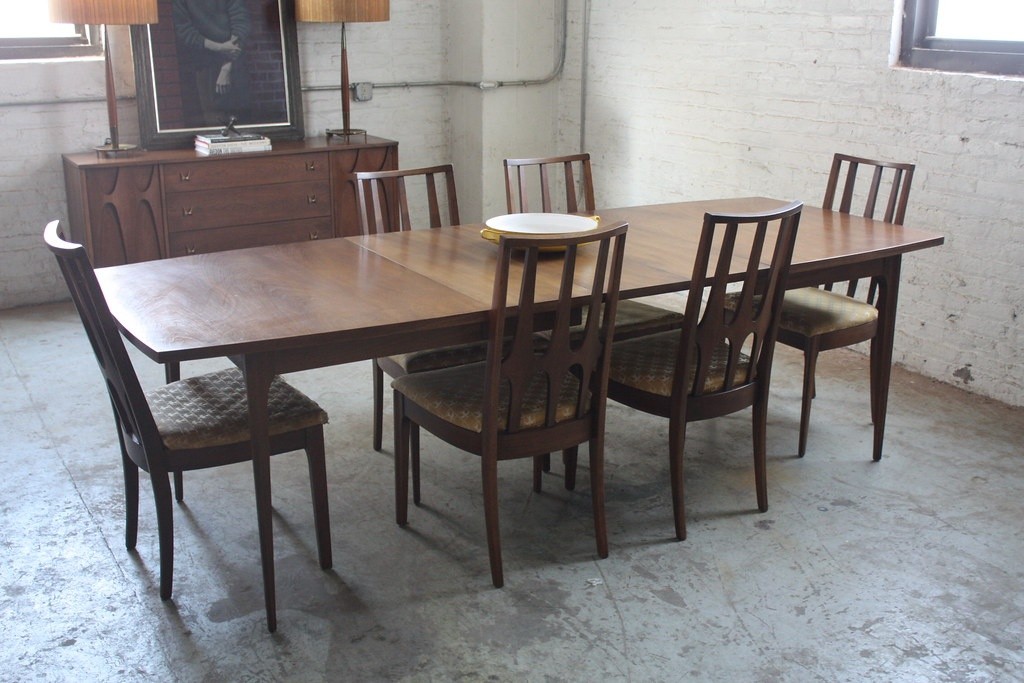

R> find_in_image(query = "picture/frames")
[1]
[127,0,304,151]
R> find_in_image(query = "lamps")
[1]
[48,0,159,160]
[294,0,390,144]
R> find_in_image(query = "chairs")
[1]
[390,220,630,589]
[41,218,332,633]
[563,199,805,543]
[503,152,683,463]
[354,162,551,506]
[723,152,917,458]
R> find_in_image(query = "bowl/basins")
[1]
[479,211,602,253]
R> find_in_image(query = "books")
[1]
[193,131,273,156]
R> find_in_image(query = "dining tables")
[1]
[91,196,944,632]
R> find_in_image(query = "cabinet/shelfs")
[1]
[60,131,399,271]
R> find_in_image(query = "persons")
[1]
[170,0,249,127]
